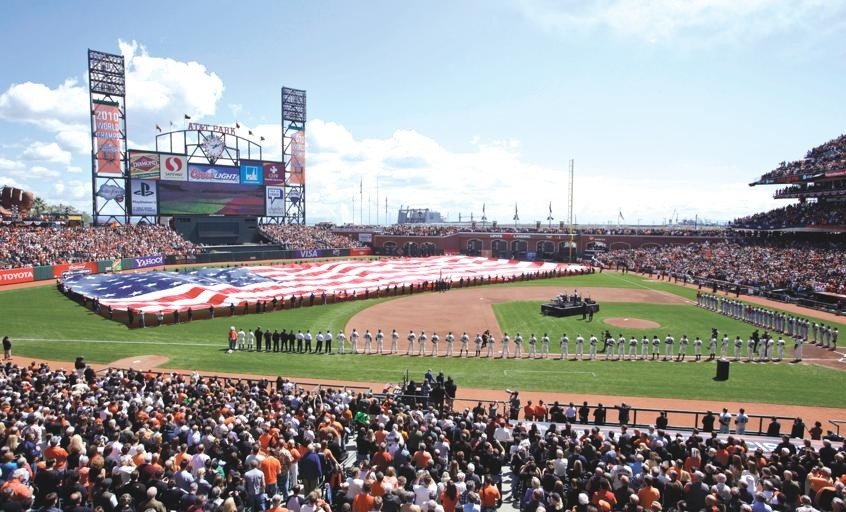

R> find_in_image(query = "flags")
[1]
[185,113,190,119]
[156,126,161,132]
[249,130,252,135]
[236,122,240,128]
[170,121,172,125]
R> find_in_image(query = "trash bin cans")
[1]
[717,358,729,378]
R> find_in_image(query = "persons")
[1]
[0,133,845,512]
